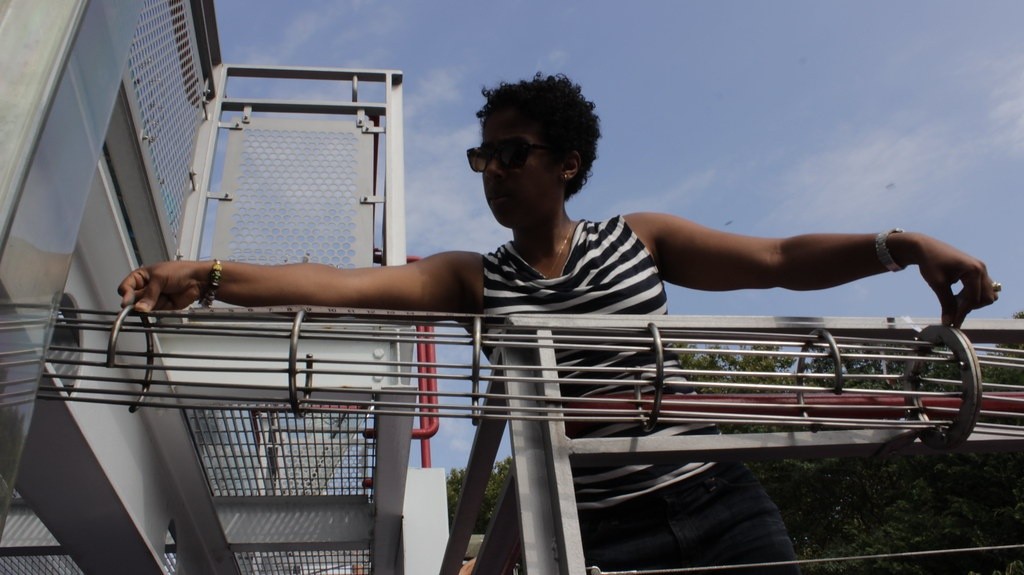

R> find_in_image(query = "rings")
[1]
[991,283,1002,291]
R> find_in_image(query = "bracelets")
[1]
[201,259,221,307]
[875,228,907,271]
[547,223,572,276]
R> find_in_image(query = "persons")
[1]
[116,72,997,574]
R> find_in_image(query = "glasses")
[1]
[467,137,563,172]
